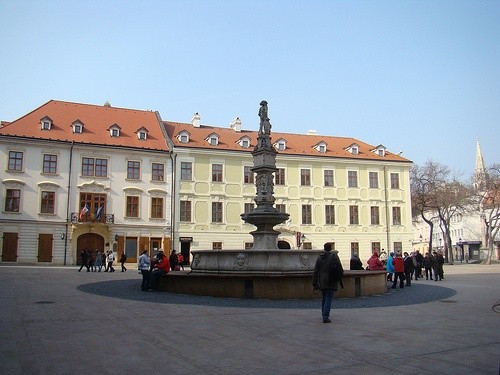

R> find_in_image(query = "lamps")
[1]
[115,234,119,241]
[61,233,65,239]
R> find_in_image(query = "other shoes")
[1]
[323,318,331,323]
[78,269,127,272]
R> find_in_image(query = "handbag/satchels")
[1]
[174,265,180,270]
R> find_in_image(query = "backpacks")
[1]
[138,257,146,270]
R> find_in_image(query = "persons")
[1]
[386,252,394,282]
[367,252,384,270]
[465,253,468,263]
[138,248,170,291]
[403,252,413,286]
[312,243,344,323]
[84,248,115,272]
[78,249,90,272]
[389,251,404,289]
[120,251,127,272]
[350,252,364,270]
[258,100,272,135]
[460,255,463,263]
[379,249,387,265]
[169,250,184,271]
[396,250,444,281]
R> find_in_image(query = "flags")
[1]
[96,205,104,221]
[79,204,90,220]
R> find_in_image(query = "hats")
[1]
[390,252,394,256]
[324,243,332,250]
[158,249,163,252]
[396,252,401,256]
[156,253,163,258]
